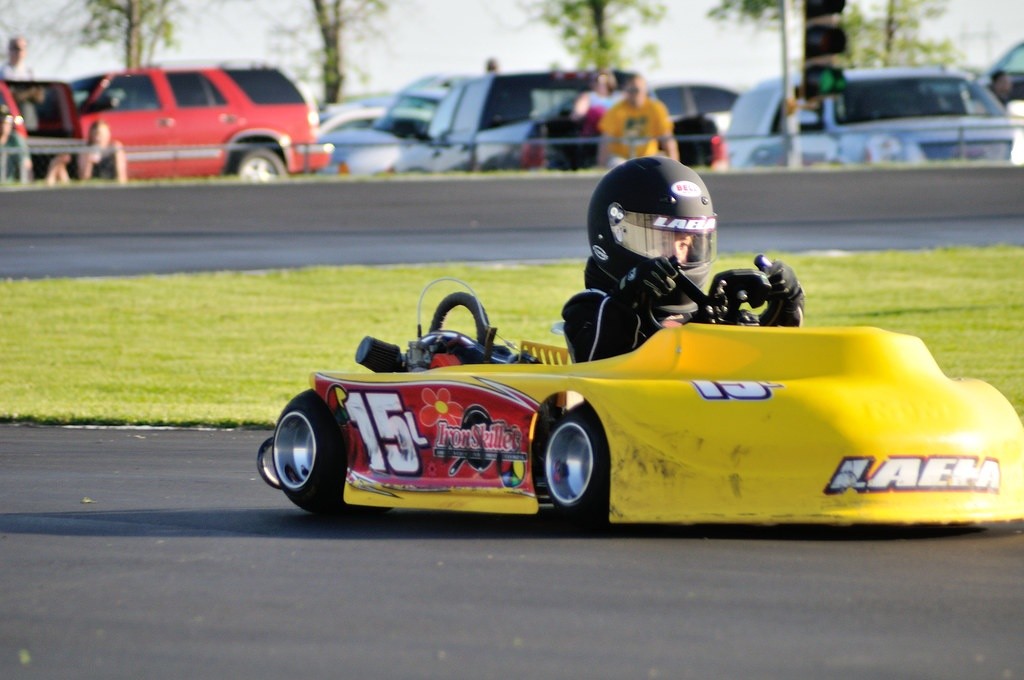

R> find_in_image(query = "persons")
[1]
[562,156,804,364]
[0,37,44,130]
[42,121,126,185]
[570,67,627,138]
[597,76,680,166]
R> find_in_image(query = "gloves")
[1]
[619,256,679,299]
[767,260,805,305]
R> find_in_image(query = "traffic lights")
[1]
[793,2,849,99]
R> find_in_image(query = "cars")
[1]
[724,68,1024,164]
[316,72,655,182]
[1,80,85,185]
[645,82,736,137]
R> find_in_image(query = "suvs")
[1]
[68,64,332,180]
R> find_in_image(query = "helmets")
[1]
[587,156,718,304]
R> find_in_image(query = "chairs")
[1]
[552,320,575,364]
